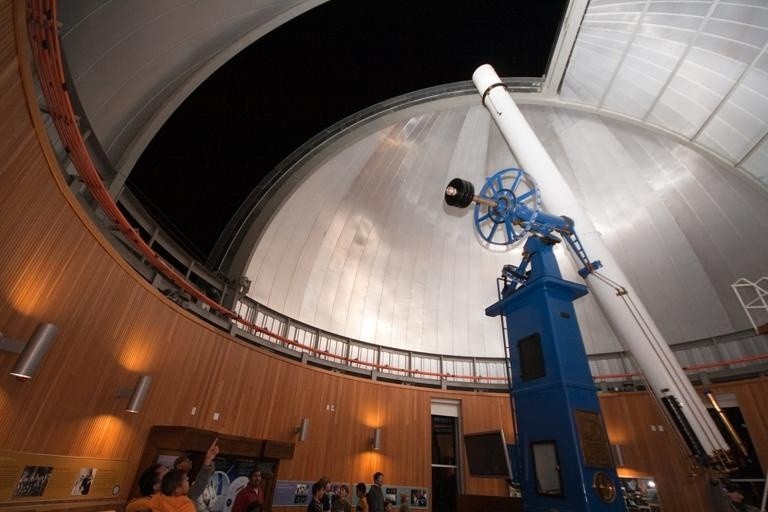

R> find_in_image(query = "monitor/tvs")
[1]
[461,429,515,482]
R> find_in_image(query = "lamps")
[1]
[369,427,380,449]
[1,321,59,381]
[115,375,151,413]
[294,417,308,443]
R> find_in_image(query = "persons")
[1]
[125,438,265,512]
[307,472,409,512]
